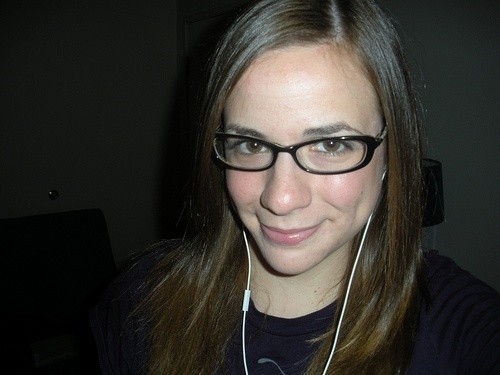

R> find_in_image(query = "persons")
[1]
[89,0,500,375]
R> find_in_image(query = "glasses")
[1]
[212,121,387,175]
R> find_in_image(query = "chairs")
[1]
[0,208,118,375]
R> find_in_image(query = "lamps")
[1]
[422,159,444,227]
